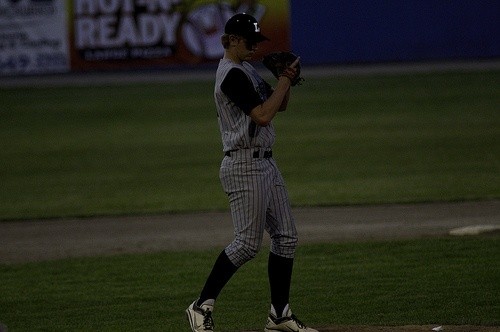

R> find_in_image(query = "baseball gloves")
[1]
[263,51,305,87]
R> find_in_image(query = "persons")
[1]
[185,13,320,331]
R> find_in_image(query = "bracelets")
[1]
[280,67,297,81]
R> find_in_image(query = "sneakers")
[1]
[185,297,216,332]
[264,307,319,332]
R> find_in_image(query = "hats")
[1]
[224,13,271,42]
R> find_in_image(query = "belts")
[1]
[224,149,273,158]
